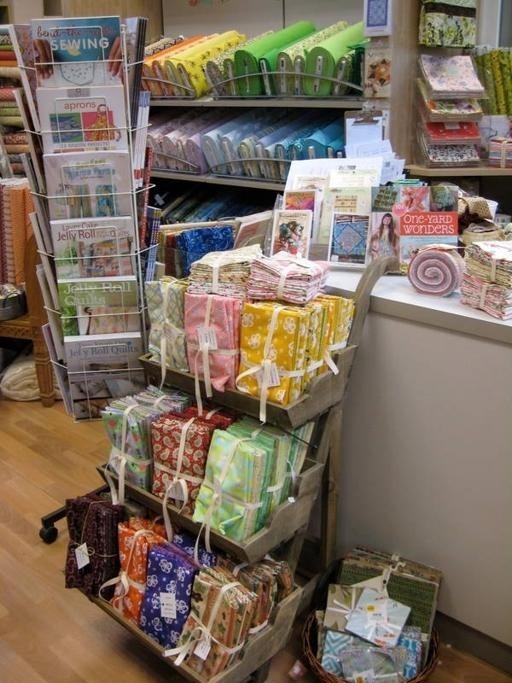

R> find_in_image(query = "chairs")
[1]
[1,234,55,409]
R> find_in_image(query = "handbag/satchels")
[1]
[1,284,27,320]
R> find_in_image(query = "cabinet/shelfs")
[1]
[40,246,391,683]
[249,265,512,674]
[139,33,390,275]
[10,22,160,423]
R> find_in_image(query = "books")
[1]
[138,147,153,250]
[50,216,139,279]
[31,16,123,86]
[35,266,66,360]
[12,87,45,194]
[41,323,73,416]
[35,86,129,154]
[133,89,150,188]
[63,332,146,421]
[9,24,42,133]
[28,212,59,310]
[57,276,143,335]
[136,205,160,296]
[20,153,55,254]
[43,150,135,219]
[124,16,148,138]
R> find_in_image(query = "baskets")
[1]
[300,604,438,683]
